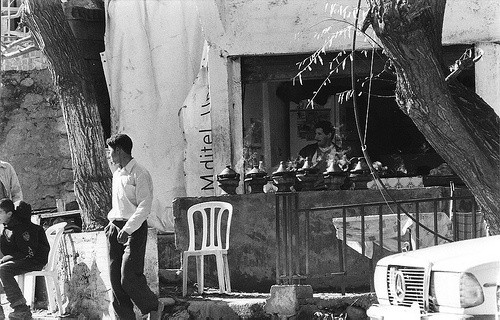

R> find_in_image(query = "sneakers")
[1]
[140,299,164,320]
[114,311,136,320]
[9,305,32,320]
[0,306,5,320]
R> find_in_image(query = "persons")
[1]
[103,134,164,320]
[0,160,50,320]
[298,121,344,168]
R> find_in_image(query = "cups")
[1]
[56,199,66,214]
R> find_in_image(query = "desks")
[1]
[332,211,454,299]
[36,210,82,233]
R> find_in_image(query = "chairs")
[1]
[14,222,67,314]
[182,201,233,297]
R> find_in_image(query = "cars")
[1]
[366,235,500,320]
